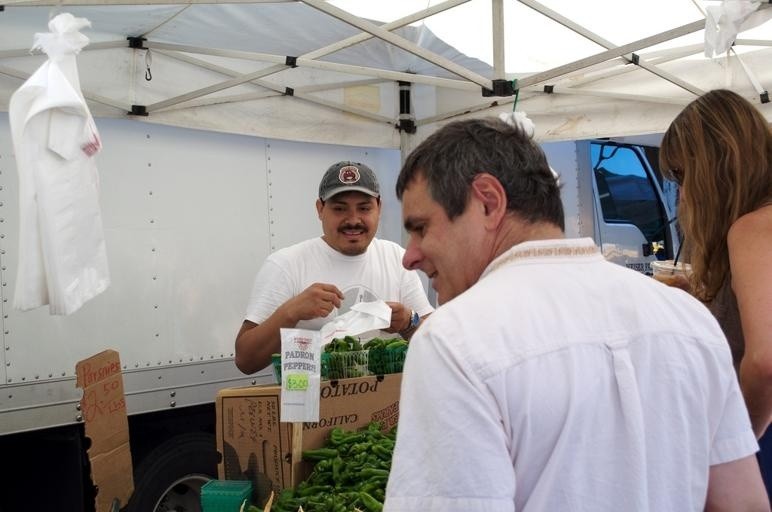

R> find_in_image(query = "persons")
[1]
[233,160,434,375]
[382,115,772,512]
[657,90,772,507]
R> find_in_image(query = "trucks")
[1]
[1,111,683,509]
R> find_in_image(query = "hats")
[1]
[319,161,381,202]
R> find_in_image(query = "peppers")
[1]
[248,337,408,512]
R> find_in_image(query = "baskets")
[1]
[271,356,281,386]
[200,479,254,512]
[321,347,408,379]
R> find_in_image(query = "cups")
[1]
[650,260,695,293]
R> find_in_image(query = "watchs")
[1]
[399,308,420,338]
